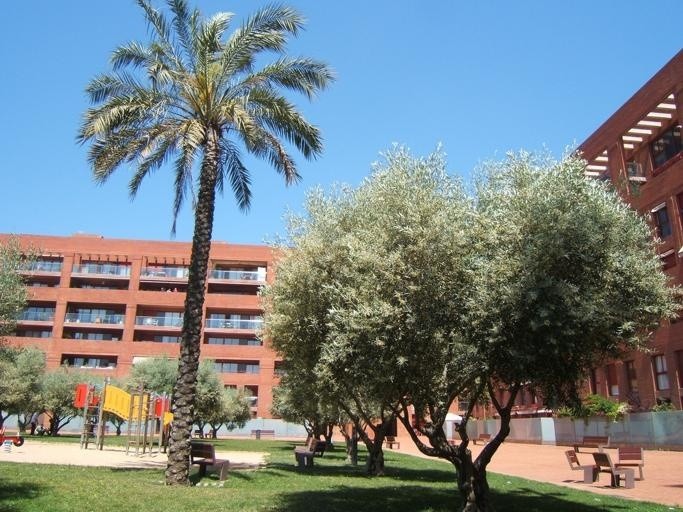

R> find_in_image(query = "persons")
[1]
[29,410,53,436]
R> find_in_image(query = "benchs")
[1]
[249,430,274,440]
[573,435,611,453]
[472,433,492,446]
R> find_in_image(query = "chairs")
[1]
[194,429,213,438]
[294,437,328,472]
[565,445,644,489]
[187,441,230,481]
